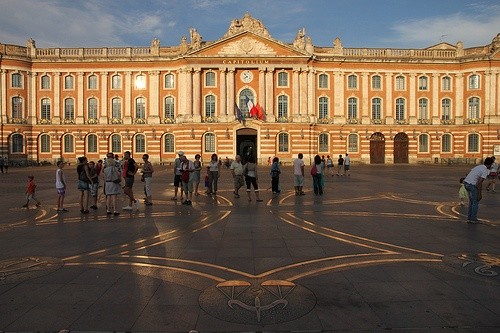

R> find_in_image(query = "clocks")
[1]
[240,70,254,83]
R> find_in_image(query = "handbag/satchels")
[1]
[244,163,248,176]
[311,163,317,176]
[79,164,91,182]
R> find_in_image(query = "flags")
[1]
[254,102,265,120]
[244,96,258,118]
[234,102,244,123]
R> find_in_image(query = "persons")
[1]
[312,155,324,197]
[269,157,281,194]
[192,153,201,196]
[292,153,306,196]
[486,155,497,192]
[21,176,41,208]
[322,152,351,177]
[203,166,211,194]
[77,152,128,216]
[171,152,185,200]
[462,158,493,224]
[180,156,195,205]
[55,160,68,212]
[141,154,154,206]
[231,155,244,198]
[0,155,10,175]
[207,154,219,195]
[458,177,467,205]
[242,154,263,202]
[123,150,140,211]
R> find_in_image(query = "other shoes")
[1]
[467,219,471,222]
[144,200,152,204]
[134,199,140,210]
[122,205,133,209]
[471,218,480,222]
[182,200,187,204]
[56,209,62,211]
[107,211,112,214]
[233,192,240,198]
[23,204,28,207]
[294,192,305,195]
[256,199,263,202]
[36,202,40,206]
[90,205,97,210]
[186,200,192,205]
[113,210,119,214]
[62,209,68,211]
[80,209,89,212]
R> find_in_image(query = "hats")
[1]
[178,150,183,155]
